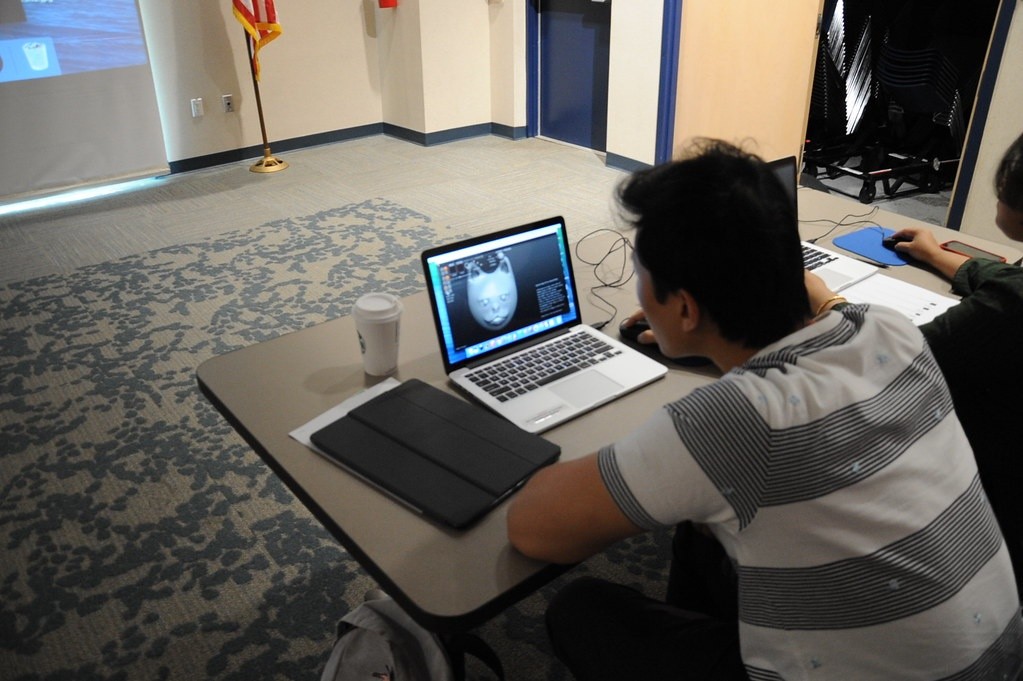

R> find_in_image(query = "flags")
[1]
[232,0,282,81]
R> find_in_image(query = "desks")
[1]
[196,181,1023,681]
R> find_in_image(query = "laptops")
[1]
[766,154,880,294]
[422,216,669,431]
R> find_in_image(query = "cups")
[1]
[352,292,403,377]
[23,43,49,70]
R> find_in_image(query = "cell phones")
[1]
[939,240,1006,265]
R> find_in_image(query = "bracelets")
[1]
[816,295,845,315]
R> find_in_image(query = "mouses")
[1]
[619,317,661,355]
[883,234,913,251]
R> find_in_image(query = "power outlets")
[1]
[191,98,205,117]
[222,94,234,112]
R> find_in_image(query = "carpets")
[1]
[0,198,682,681]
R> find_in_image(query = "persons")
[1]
[507,142,1023,681]
[803,130,1023,497]
